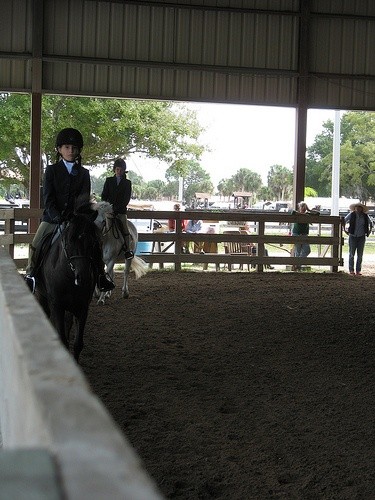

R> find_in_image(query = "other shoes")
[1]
[357,271,362,275]
[350,271,355,274]
[266,265,274,269]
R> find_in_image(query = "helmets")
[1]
[57,128,83,148]
[114,159,126,170]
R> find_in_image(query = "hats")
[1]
[350,203,368,212]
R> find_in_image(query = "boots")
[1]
[123,234,133,258]
[93,241,116,290]
[24,244,38,293]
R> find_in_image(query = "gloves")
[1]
[60,206,73,219]
[51,216,61,225]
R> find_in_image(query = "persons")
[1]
[168,204,205,253]
[343,203,373,275]
[102,158,132,258]
[26,128,114,291]
[240,227,275,269]
[291,202,321,271]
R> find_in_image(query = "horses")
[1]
[92,201,150,306]
[25,195,105,363]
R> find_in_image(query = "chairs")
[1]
[223,230,250,272]
[240,231,268,269]
